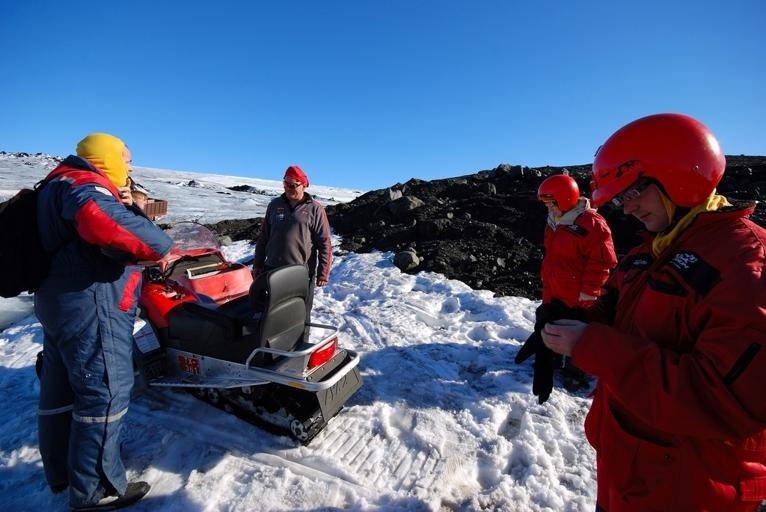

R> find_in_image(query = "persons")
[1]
[530,174,619,375]
[33,132,173,512]
[251,165,333,343]
[542,112,766,512]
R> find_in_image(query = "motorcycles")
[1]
[118,213,365,446]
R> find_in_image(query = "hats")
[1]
[77,132,129,187]
[284,165,309,187]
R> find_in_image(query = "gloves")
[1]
[512,296,590,405]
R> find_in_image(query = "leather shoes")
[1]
[71,481,152,511]
[49,467,72,494]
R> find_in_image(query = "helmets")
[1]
[536,174,581,213]
[589,111,728,210]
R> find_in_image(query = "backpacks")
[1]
[0,166,92,297]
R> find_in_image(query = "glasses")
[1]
[610,179,652,209]
[283,180,303,189]
[542,200,558,209]
